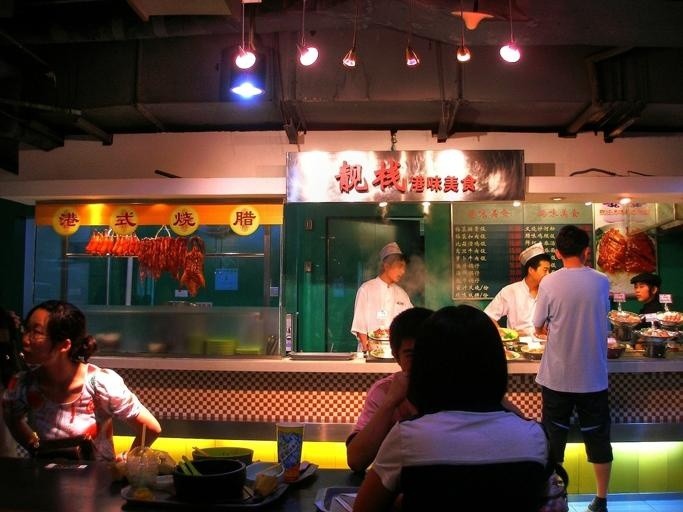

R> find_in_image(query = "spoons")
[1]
[182,455,203,476]
[178,461,191,475]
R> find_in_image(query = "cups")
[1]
[125,447,160,502]
[275,422,305,482]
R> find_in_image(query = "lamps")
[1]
[498,6,526,66]
[294,0,318,66]
[453,0,473,62]
[228,71,268,98]
[231,2,260,72]
[344,1,361,69]
[402,2,423,68]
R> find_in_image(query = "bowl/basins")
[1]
[192,448,253,467]
[246,462,284,483]
[172,458,247,504]
[607,344,625,358]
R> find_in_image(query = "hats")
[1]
[519,241,545,265]
[630,273,659,285]
[379,241,401,261]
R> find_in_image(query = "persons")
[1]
[483,243,552,336]
[351,242,414,352]
[0,299,161,463]
[532,224,614,512]
[352,304,549,511]
[346,306,525,474]
[630,272,665,329]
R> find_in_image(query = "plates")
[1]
[606,310,640,328]
[530,331,547,344]
[187,336,204,355]
[204,338,235,356]
[638,327,677,344]
[497,327,519,342]
[370,348,395,361]
[367,329,389,341]
[236,345,261,355]
[521,345,544,360]
[656,311,682,327]
[505,350,520,362]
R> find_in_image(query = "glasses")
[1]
[20,322,47,339]
